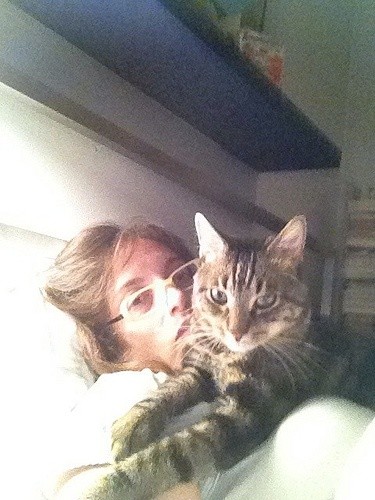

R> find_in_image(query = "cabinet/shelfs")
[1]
[11,0,342,173]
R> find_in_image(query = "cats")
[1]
[87,210,375,500]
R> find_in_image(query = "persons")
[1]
[42,223,200,500]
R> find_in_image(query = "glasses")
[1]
[104,256,202,327]
